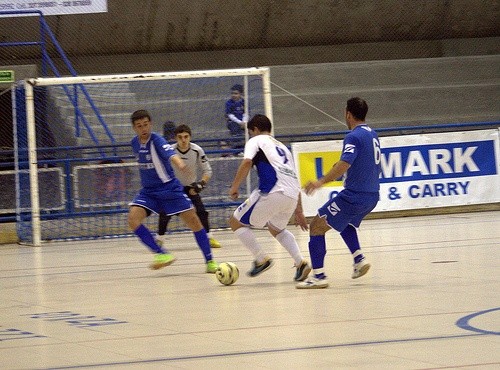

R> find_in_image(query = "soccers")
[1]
[216,262,239,285]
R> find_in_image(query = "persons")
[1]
[296,96,383,291]
[223,84,249,138]
[156,121,225,250]
[227,113,312,281]
[127,110,222,273]
